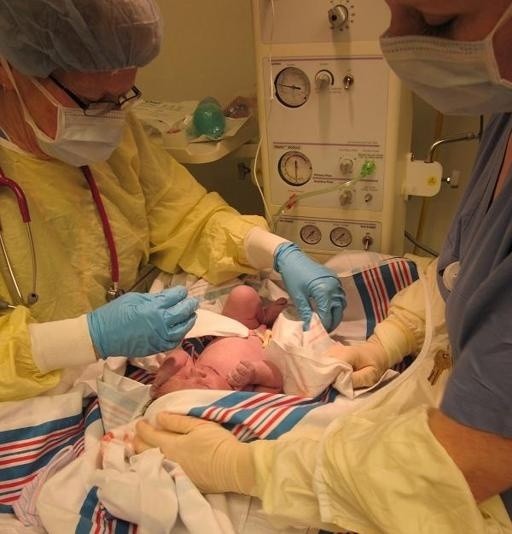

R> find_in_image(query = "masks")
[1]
[378,4,512,117]
[15,76,125,168]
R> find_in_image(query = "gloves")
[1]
[275,242,347,334]
[86,285,200,359]
[134,414,249,495]
[325,338,389,389]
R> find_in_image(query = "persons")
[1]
[0,0,346,401]
[134,0,512,533]
[150,285,288,399]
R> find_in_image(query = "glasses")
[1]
[49,75,142,117]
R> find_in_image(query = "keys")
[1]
[427,349,452,385]
